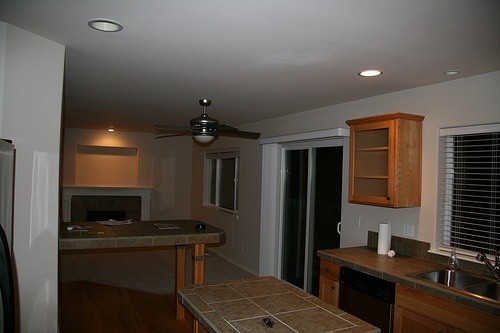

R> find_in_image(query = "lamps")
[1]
[192,132,218,144]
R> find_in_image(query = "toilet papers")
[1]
[378,223,392,255]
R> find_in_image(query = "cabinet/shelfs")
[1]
[319,257,343,307]
[345,112,425,209]
[393,283,500,333]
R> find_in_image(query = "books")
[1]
[153,224,179,229]
[72,226,88,231]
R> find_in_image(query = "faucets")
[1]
[475,252,500,283]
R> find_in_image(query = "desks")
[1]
[59,219,226,333]
[178,275,382,333]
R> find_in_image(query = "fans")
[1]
[154,98,261,140]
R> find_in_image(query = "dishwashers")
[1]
[338,266,396,333]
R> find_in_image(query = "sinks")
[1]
[414,267,495,289]
[462,283,500,301]
